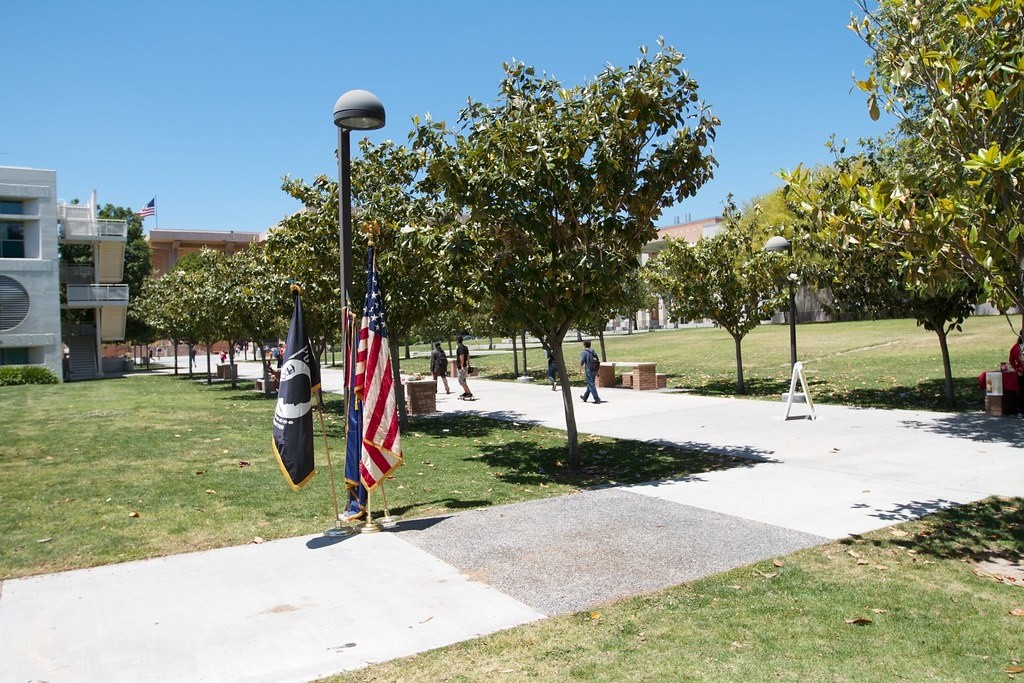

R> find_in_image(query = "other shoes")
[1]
[593,401,600,404]
[446,388,449,394]
[580,395,586,402]
[553,382,556,390]
[459,393,472,398]
[195,365,196,367]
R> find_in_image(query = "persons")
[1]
[456,336,473,397]
[430,342,450,394]
[156,345,161,359]
[579,339,601,404]
[147,347,155,361]
[277,344,287,368]
[544,345,558,391]
[219,349,227,363]
[191,345,196,367]
[1009,329,1024,420]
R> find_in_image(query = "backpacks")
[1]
[584,349,600,371]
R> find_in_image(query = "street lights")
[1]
[764,235,807,404]
[331,88,387,525]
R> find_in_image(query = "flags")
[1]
[354,247,403,495]
[342,314,368,520]
[271,286,322,491]
[138,198,155,218]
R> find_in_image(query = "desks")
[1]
[217,363,238,379]
[392,374,437,415]
[273,368,282,390]
[595,362,657,391]
[447,358,458,377]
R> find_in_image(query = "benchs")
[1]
[466,365,478,377]
[621,372,667,389]
[255,378,274,392]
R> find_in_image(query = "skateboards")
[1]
[457,394,475,401]
